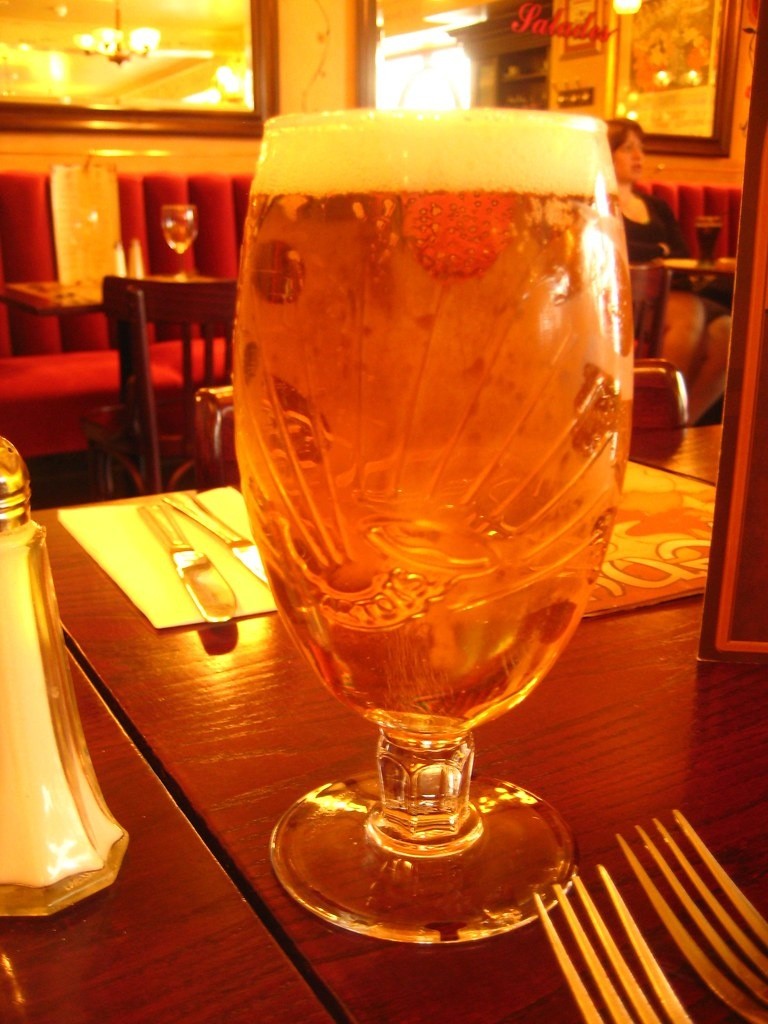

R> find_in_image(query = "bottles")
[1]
[130,238,144,280]
[0,435,130,917]
[113,241,126,277]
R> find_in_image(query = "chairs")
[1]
[80,275,237,501]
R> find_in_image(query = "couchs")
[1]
[0,170,258,460]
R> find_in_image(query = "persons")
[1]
[606,118,732,427]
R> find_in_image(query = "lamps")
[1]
[612,0,642,15]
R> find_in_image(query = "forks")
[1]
[533,864,694,1024]
[614,809,768,1024]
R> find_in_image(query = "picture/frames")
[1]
[604,0,743,157]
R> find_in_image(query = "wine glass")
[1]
[227,116,633,946]
[163,204,198,282]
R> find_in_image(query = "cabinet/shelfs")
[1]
[445,3,551,109]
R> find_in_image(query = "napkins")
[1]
[56,485,278,630]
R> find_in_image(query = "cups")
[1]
[696,216,721,267]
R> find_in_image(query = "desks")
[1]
[31,457,767,1024]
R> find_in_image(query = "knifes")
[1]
[162,494,268,584]
[141,502,236,623]
